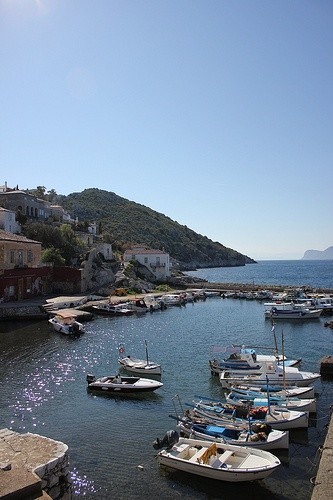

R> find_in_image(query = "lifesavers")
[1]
[119,347,124,354]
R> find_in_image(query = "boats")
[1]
[85,373,165,399]
[219,288,333,313]
[118,339,163,375]
[152,424,283,483]
[263,301,324,319]
[47,311,86,338]
[190,322,323,430]
[167,393,290,451]
[91,290,206,316]
